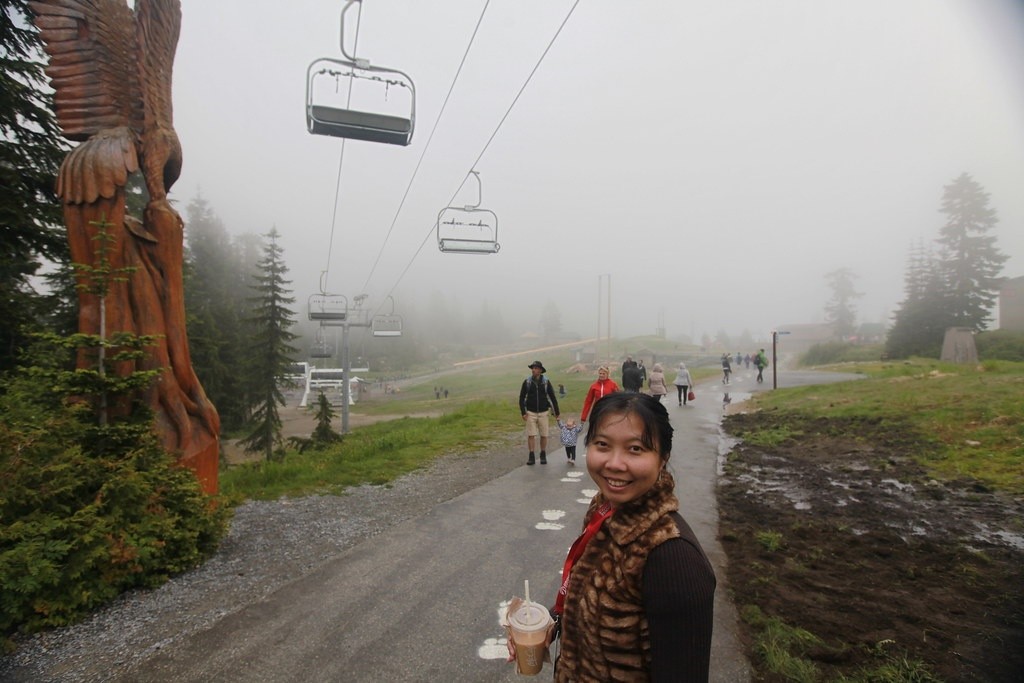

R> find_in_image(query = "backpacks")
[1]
[753,354,762,364]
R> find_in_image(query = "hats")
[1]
[529,361,546,373]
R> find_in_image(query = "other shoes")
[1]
[571,460,574,465]
[679,403,681,406]
[684,402,686,404]
[567,459,571,463]
[538,454,548,465]
[527,455,535,465]
[723,380,725,384]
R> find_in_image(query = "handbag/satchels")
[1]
[688,386,695,401]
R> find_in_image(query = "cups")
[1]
[507,602,552,676]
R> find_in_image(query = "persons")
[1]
[362,373,414,395]
[648,363,668,402]
[581,365,621,425]
[673,363,693,406]
[556,416,585,465]
[433,385,449,399]
[622,355,647,392]
[721,349,767,384]
[435,360,449,372]
[506,391,716,683]
[518,361,559,464]
